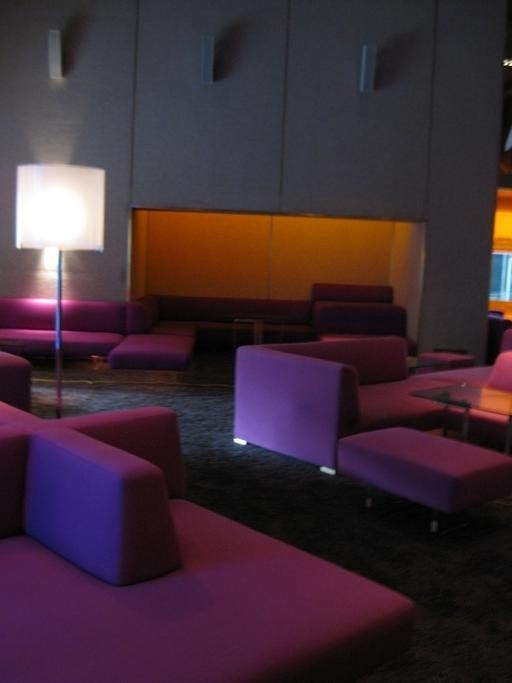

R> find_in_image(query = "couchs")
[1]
[0,282,512,678]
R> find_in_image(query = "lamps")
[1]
[13,161,107,417]
